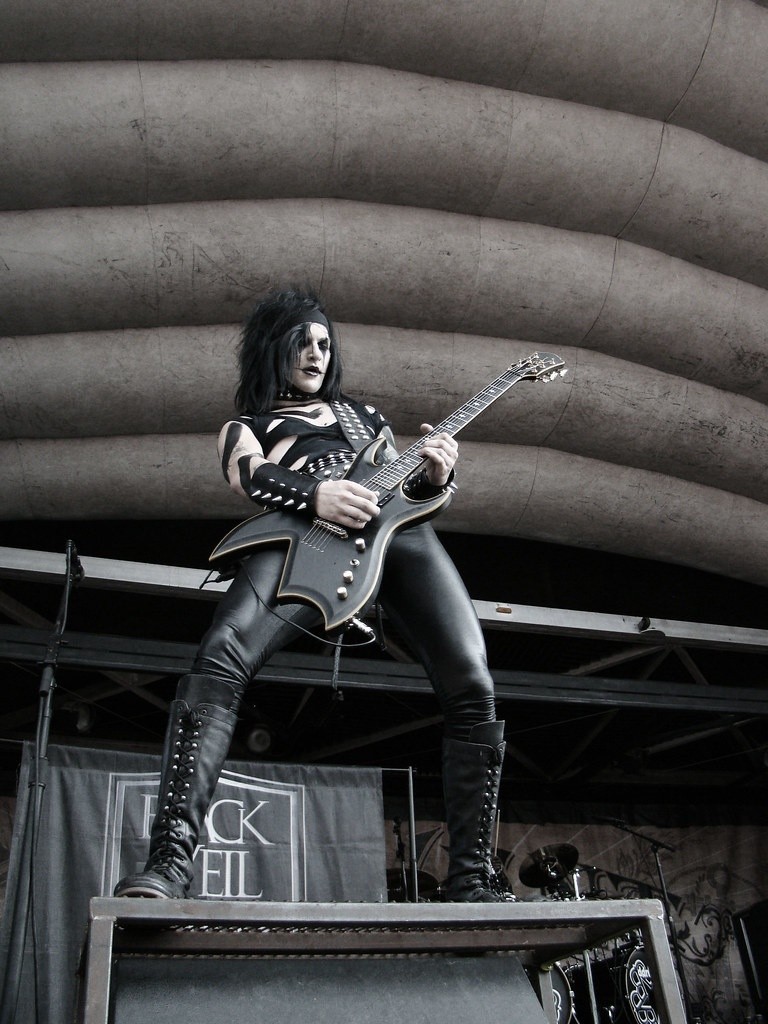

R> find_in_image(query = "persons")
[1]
[114,289,518,904]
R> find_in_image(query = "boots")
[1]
[438,720,522,903]
[113,674,243,899]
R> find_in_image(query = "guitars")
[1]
[206,349,570,639]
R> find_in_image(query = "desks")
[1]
[76,895,686,1024]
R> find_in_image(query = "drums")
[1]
[561,945,662,1024]
[523,961,575,1024]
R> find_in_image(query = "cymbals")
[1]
[517,842,580,889]
[386,867,440,903]
